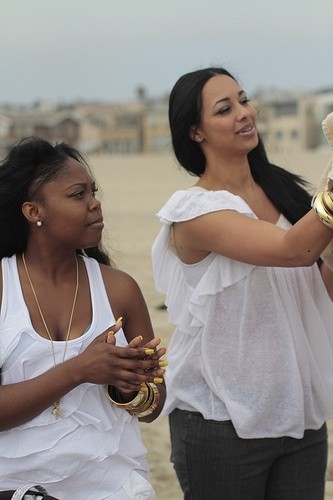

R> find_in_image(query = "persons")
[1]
[150,68,333,500]
[0,134,169,500]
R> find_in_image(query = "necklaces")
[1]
[23,254,79,417]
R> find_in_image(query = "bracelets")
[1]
[107,383,163,419]
[312,189,333,228]
